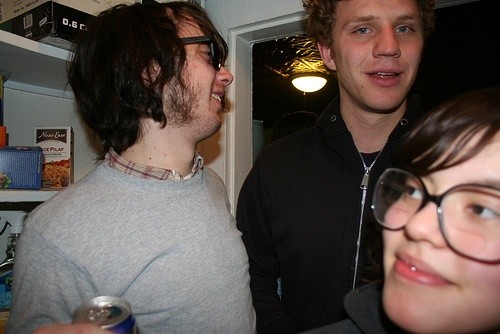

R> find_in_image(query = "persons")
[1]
[236,0,447,334]
[8,1,256,334]
[299,75,500,334]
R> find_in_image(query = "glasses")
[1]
[372,167,500,264]
[178,32,224,71]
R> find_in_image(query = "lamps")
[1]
[252,35,331,92]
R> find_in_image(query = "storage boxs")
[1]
[0,0,144,53]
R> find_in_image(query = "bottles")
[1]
[0,226,24,311]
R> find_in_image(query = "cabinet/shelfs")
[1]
[0,28,113,202]
